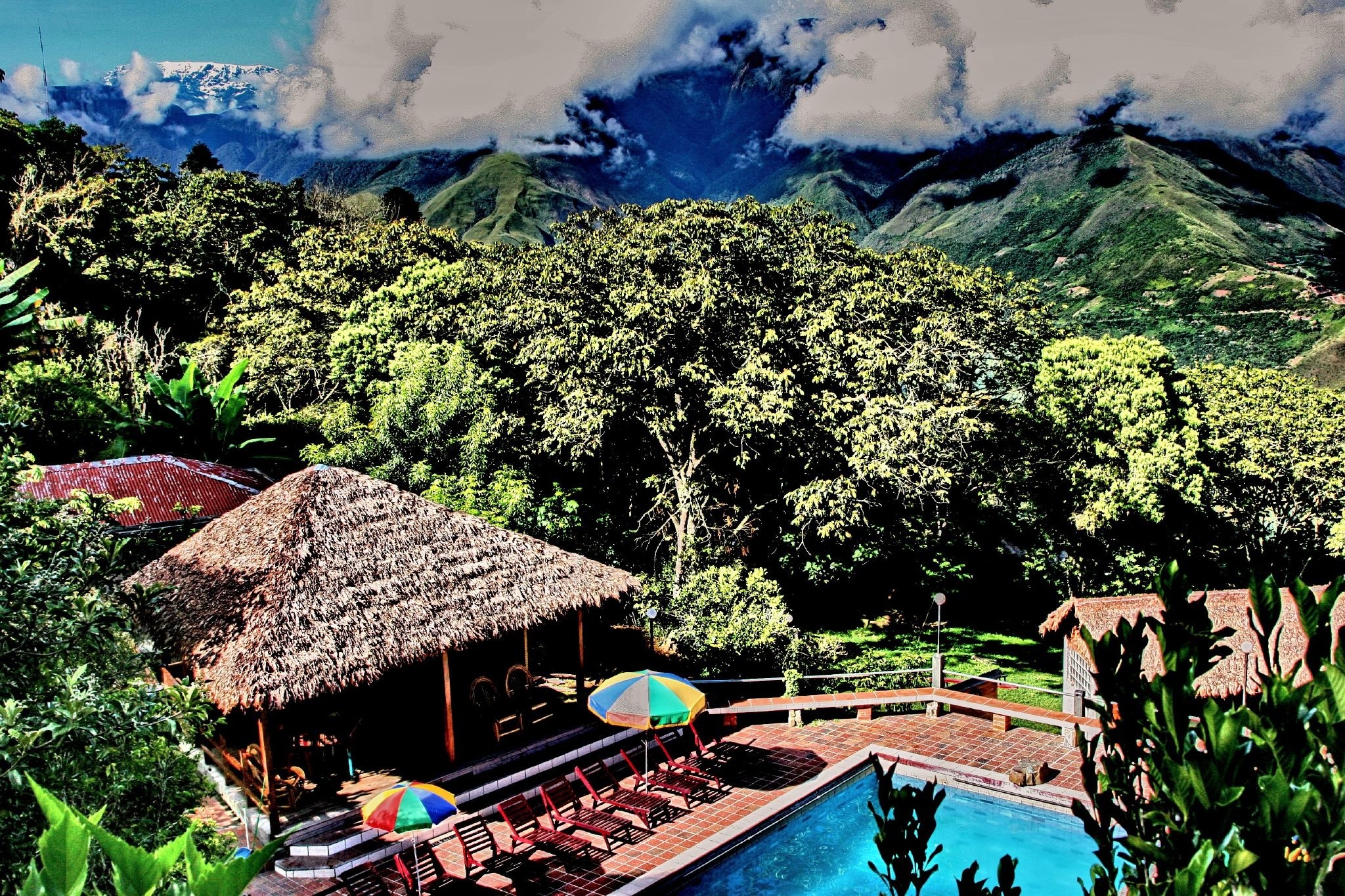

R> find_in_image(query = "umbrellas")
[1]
[360,779,457,896]
[587,669,707,796]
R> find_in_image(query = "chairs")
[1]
[470,676,523,742]
[505,664,553,724]
[338,710,757,896]
[241,749,295,808]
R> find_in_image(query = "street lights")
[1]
[646,608,659,652]
[935,593,947,654]
